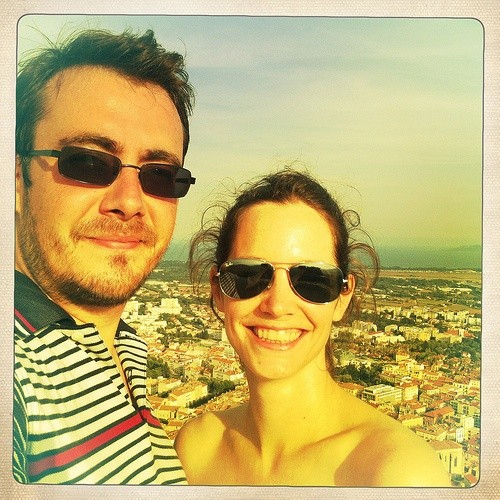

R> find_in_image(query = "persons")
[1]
[17,23,197,486]
[173,167,454,486]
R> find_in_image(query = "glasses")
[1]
[213,258,348,305]
[21,145,195,199]
